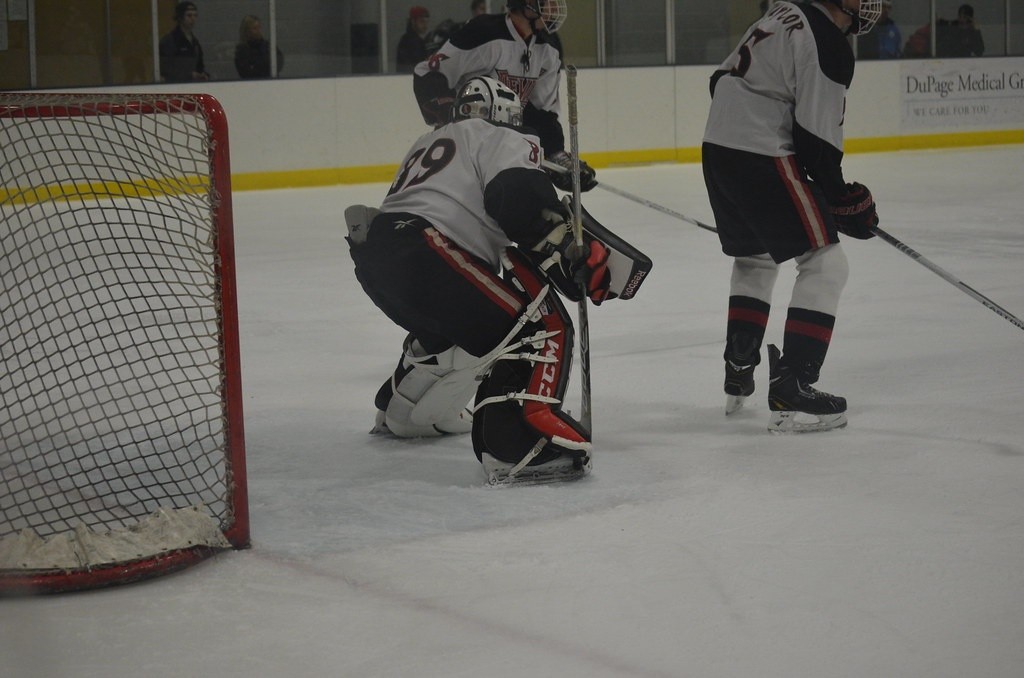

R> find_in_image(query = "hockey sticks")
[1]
[870,223,1024,327]
[564,63,589,481]
[542,161,717,234]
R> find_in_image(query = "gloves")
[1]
[545,151,598,191]
[827,182,879,239]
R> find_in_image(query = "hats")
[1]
[174,2,197,17]
[409,7,430,20]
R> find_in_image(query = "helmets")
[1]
[451,75,523,129]
[839,0,882,36]
[505,0,568,36]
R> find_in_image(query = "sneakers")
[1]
[765,342,848,431]
[724,355,757,414]
[374,378,399,431]
[483,443,588,484]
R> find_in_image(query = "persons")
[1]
[701,0,882,413]
[159,2,209,83]
[350,0,610,466]
[234,15,284,79]
[856,0,984,59]
